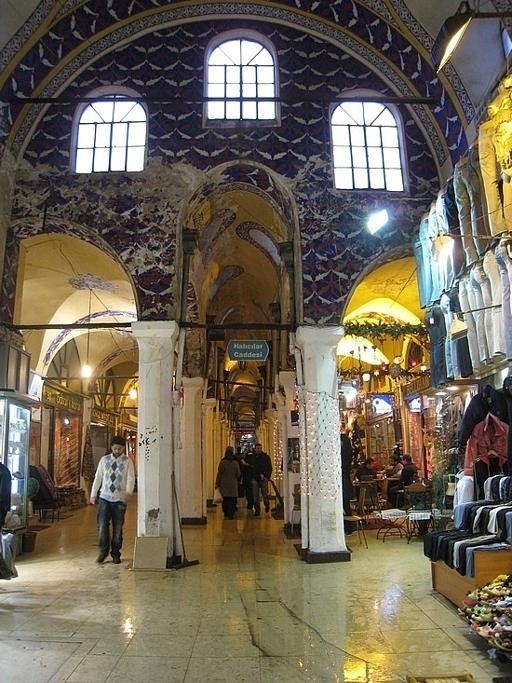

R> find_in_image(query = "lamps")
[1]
[447,304,502,343]
[79,288,97,379]
[429,0,511,78]
[433,229,512,253]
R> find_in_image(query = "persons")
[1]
[90,436,135,564]
[0,462,11,532]
[215,444,272,520]
[340,434,417,536]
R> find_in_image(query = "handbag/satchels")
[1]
[0,533,18,581]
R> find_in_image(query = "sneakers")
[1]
[458,574,510,652]
[96,552,120,564]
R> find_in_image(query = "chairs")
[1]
[343,482,437,548]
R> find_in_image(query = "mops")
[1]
[170,474,200,569]
[166,472,182,569]
